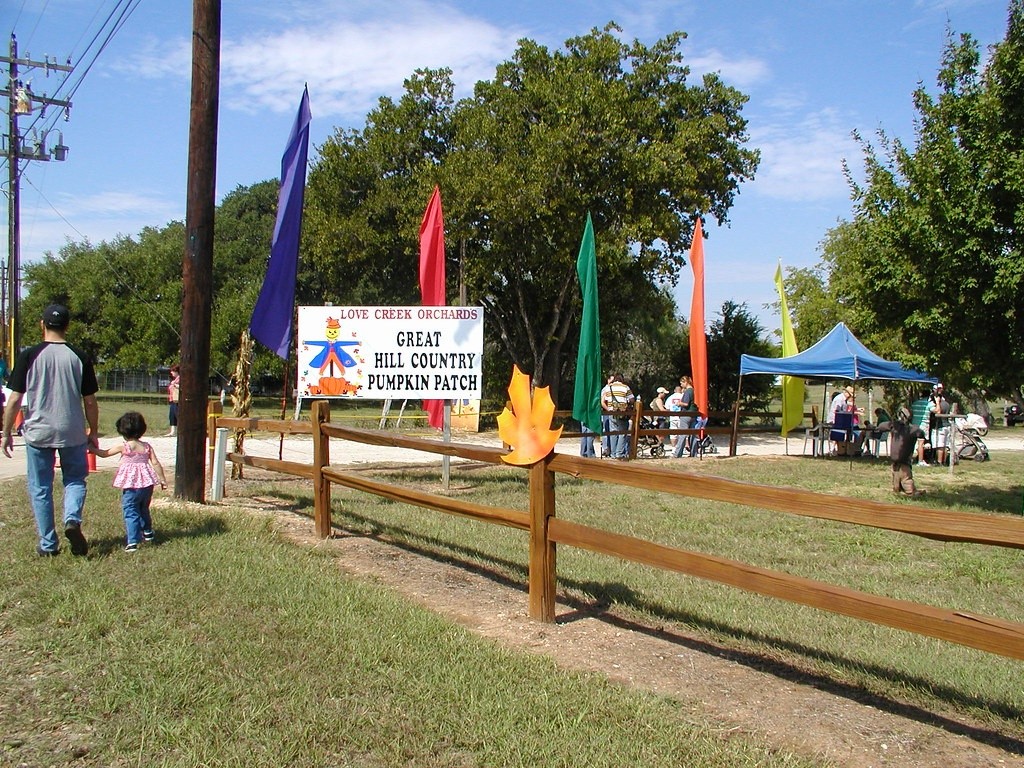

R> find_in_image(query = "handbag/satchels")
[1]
[173,387,179,403]
[613,402,631,421]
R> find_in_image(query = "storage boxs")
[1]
[829,429,846,442]
[838,440,860,456]
[834,413,853,425]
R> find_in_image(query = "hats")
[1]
[657,387,669,393]
[43,304,70,326]
[842,386,856,398]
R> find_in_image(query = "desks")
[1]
[822,426,881,458]
[935,414,969,474]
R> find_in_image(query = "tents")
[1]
[730,322,940,457]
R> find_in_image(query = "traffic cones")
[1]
[15,410,24,429]
[85,428,102,474]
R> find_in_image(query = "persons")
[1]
[910,428,931,465]
[580,373,708,458]
[220,389,227,406]
[665,376,697,458]
[828,386,893,456]
[605,373,635,461]
[88,412,168,553]
[1,304,98,557]
[580,420,597,458]
[650,388,669,447]
[916,383,950,466]
[163,365,182,437]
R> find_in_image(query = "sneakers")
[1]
[917,460,930,466]
[125,543,137,552]
[65,521,88,556]
[142,529,156,540]
[931,461,947,467]
[37,546,55,556]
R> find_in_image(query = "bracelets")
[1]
[160,480,166,483]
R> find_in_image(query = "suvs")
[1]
[1005,402,1023,427]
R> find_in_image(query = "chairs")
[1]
[862,422,894,458]
[802,423,833,458]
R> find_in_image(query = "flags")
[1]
[572,211,602,439]
[688,218,708,420]
[249,83,313,361]
[774,257,805,437]
[419,184,446,429]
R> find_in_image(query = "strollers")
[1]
[957,413,990,462]
[686,430,717,454]
[630,407,666,458]
